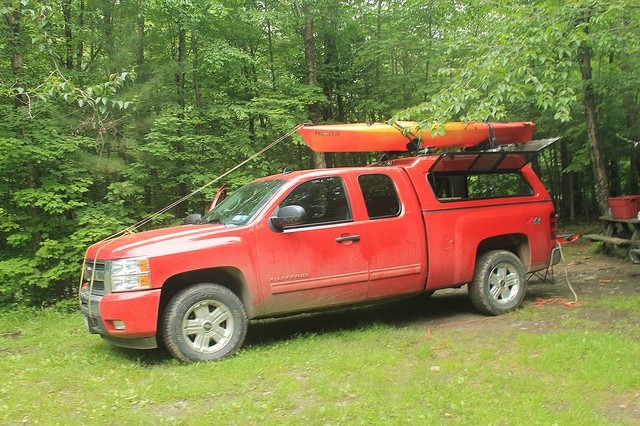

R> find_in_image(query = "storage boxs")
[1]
[608,195,640,219]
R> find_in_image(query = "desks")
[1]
[599,215,640,256]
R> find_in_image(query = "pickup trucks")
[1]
[77,136,562,363]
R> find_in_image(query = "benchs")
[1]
[582,234,631,246]
